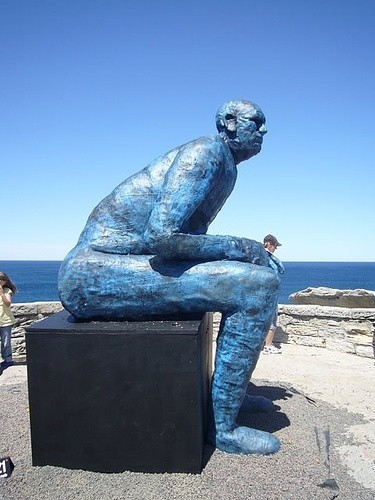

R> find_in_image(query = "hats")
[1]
[264,234,282,246]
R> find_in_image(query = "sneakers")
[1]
[262,345,282,353]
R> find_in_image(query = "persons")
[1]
[56,98,280,456]
[0,271,18,366]
[261,234,282,355]
